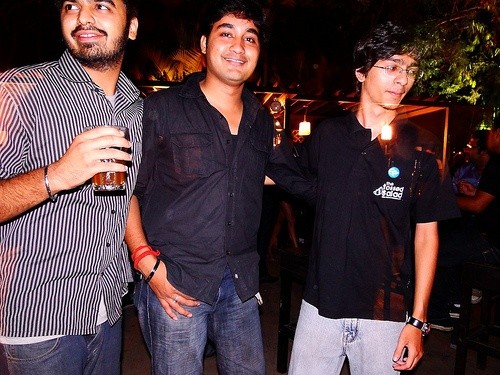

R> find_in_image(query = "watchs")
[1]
[407,318,431,336]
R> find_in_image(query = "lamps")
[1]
[299,114,311,136]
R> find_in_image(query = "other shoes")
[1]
[471,287,483,304]
[427,316,454,332]
[449,302,461,318]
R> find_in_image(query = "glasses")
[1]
[372,64,419,77]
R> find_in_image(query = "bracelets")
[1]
[145,258,160,284]
[44,165,57,201]
[132,245,160,269]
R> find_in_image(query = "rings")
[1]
[174,296,179,301]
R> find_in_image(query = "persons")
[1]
[123,0,313,375]
[268,129,301,254]
[265,23,439,375]
[398,112,500,331]
[0,0,143,375]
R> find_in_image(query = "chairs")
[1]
[454,262,500,375]
[271,185,315,374]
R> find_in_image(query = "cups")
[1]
[83,126,130,192]
